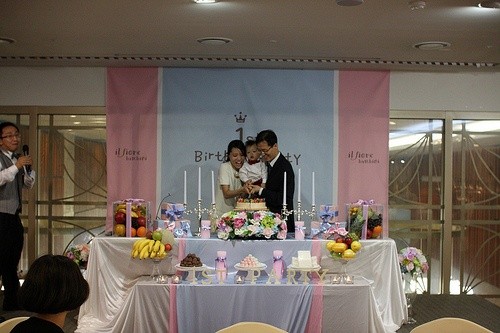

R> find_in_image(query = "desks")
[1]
[134,275,372,333]
[87,229,408,333]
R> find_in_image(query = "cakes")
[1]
[236,198,266,209]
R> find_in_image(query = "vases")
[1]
[401,271,416,325]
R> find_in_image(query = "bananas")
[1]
[131,238,165,259]
[114,204,139,218]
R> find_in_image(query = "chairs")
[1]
[0,316,30,333]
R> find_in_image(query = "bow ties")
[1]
[11,152,21,160]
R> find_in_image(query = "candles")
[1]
[298,166,301,201]
[215,250,227,280]
[282,172,287,205]
[272,250,283,280]
[198,167,202,200]
[201,219,211,239]
[312,171,316,205]
[211,170,216,203]
[183,170,187,204]
[295,220,306,240]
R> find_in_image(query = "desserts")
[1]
[240,254,259,267]
[180,253,202,267]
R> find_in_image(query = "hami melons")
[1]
[160,229,174,244]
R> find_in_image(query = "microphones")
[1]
[22,144,31,174]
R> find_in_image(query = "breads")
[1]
[291,250,317,268]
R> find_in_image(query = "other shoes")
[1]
[2,295,22,311]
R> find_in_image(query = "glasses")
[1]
[257,143,275,154]
[2,131,21,139]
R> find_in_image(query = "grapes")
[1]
[345,233,359,241]
[367,213,383,231]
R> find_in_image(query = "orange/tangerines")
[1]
[326,240,361,259]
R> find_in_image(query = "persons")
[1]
[237,140,267,200]
[249,129,297,234]
[214,139,251,225]
[0,121,35,295]
[7,254,92,333]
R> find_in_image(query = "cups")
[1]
[234,275,245,284]
[156,274,169,284]
[343,275,356,284]
[330,274,342,284]
[171,275,183,284]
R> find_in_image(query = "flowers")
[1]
[397,246,429,277]
[213,209,288,241]
[64,243,92,269]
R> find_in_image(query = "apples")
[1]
[114,212,147,236]
[367,226,381,239]
[165,244,172,251]
[335,238,352,245]
[146,231,162,240]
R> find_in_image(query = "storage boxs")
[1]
[344,199,383,240]
[160,202,183,220]
[319,203,339,223]
[173,219,191,238]
[153,219,176,237]
[328,222,347,240]
[310,220,327,240]
[111,198,151,238]
